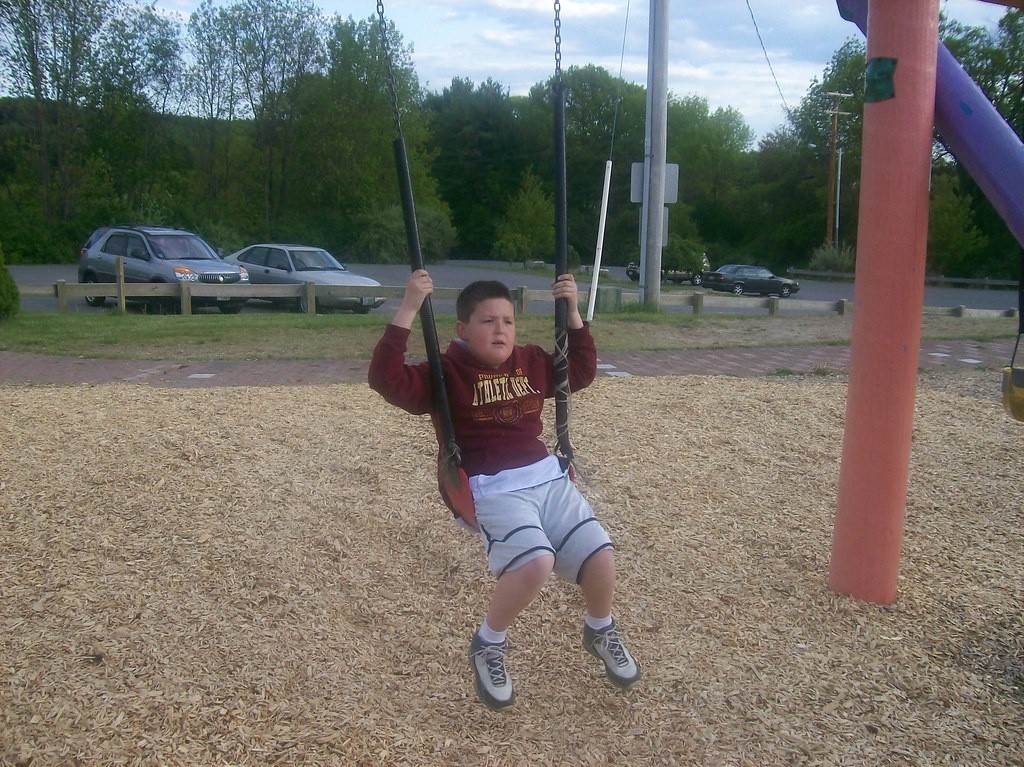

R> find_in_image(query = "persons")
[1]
[368,269,642,711]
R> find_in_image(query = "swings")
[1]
[378,35,579,537]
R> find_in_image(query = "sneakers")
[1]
[469,630,514,711]
[582,615,641,690]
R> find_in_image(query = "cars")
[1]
[224,243,389,313]
[701,264,800,299]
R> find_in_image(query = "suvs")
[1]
[78,224,251,315]
[626,253,711,286]
